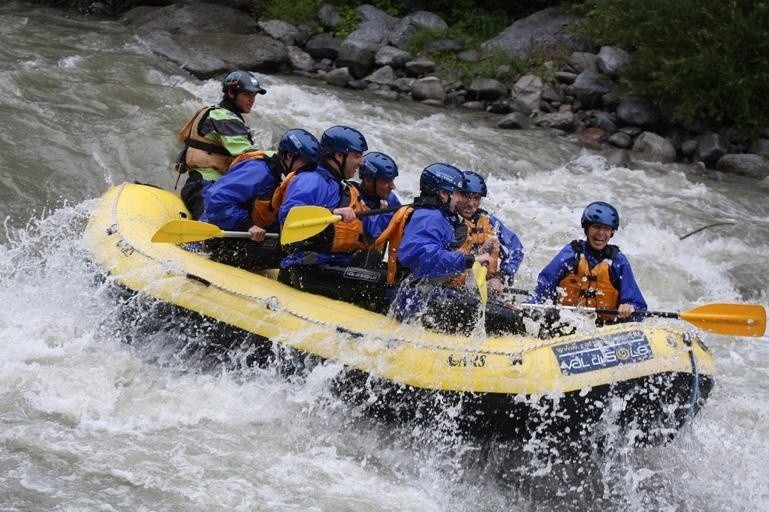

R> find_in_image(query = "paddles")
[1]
[506,301,766,337]
[151,219,279,244]
[280,204,408,245]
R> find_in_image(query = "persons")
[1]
[520,201,648,341]
[181,69,267,221]
[395,162,524,337]
[278,125,411,313]
[198,128,321,272]
[447,171,526,301]
[337,151,403,265]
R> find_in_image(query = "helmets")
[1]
[360,152,398,178]
[320,125,368,158]
[224,70,266,95]
[420,163,487,197]
[279,129,320,162]
[581,202,619,230]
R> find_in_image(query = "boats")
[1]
[82,184,714,447]
